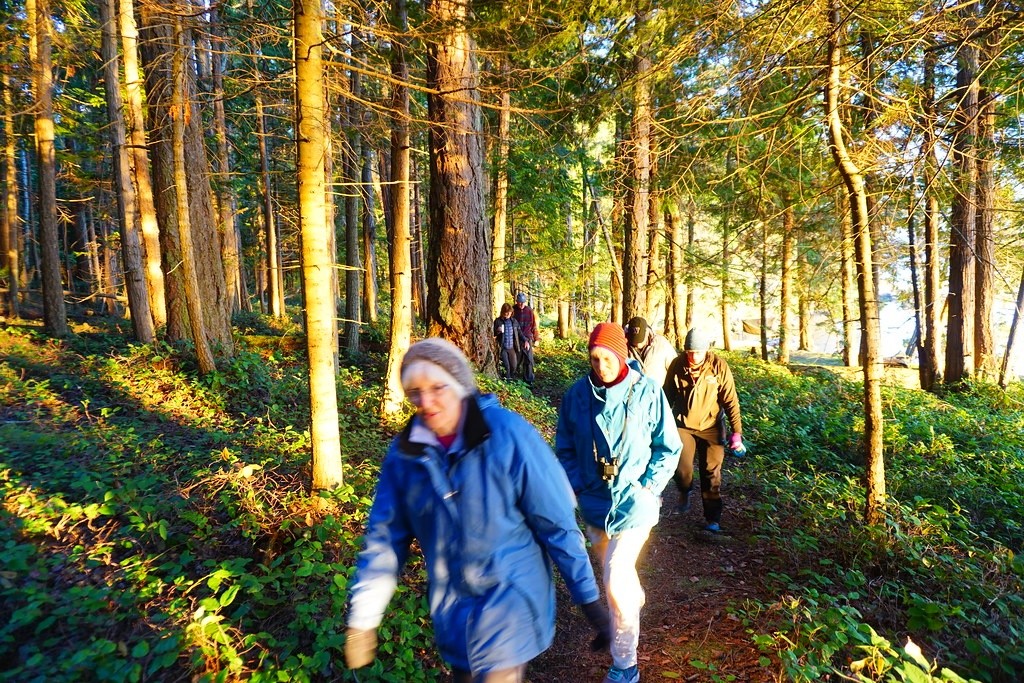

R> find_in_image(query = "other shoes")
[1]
[673,490,692,514]
[706,520,719,531]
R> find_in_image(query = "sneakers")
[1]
[602,665,640,683]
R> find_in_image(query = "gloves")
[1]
[730,433,743,449]
[583,601,610,652]
[345,627,377,669]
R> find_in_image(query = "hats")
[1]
[684,327,709,353]
[626,317,647,343]
[400,337,475,401]
[515,293,527,303]
[590,322,628,359]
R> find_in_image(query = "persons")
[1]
[511,292,541,382]
[663,326,743,532]
[343,335,614,683]
[553,317,684,683]
[492,302,530,382]
